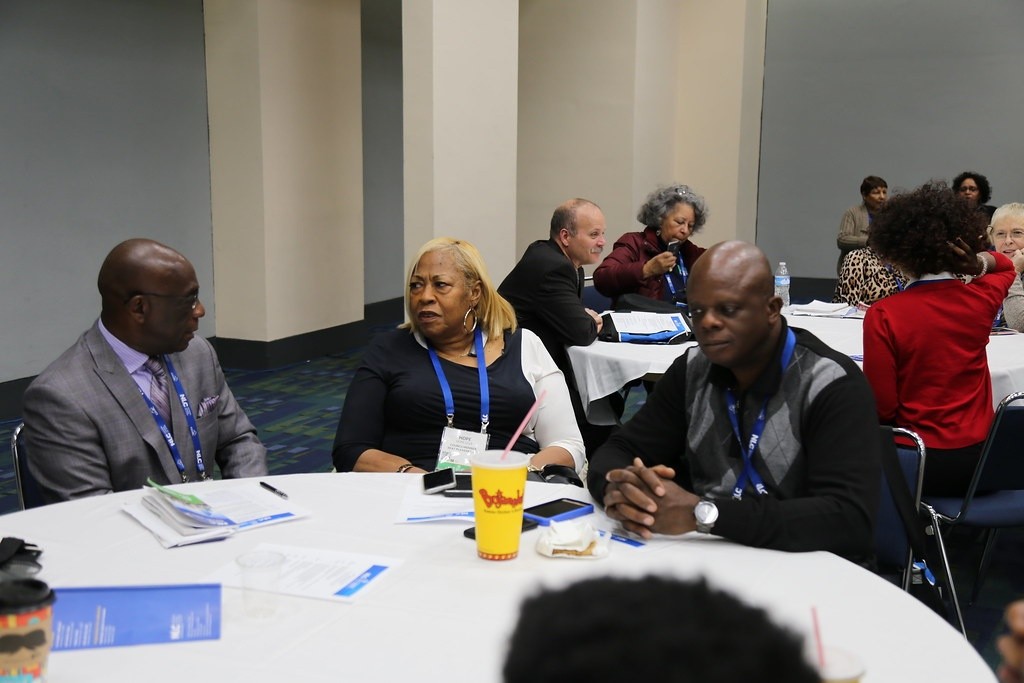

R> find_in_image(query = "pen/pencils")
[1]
[259,482,288,499]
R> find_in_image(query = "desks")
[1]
[568,303,1024,405]
[0,471,998,683]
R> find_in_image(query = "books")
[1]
[140,476,238,538]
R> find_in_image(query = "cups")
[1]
[468,450,530,560]
[0,577,58,683]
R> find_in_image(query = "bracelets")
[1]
[397,463,413,473]
[971,253,988,279]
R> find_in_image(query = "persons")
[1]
[836,171,1024,332]
[502,570,824,683]
[993,599,1024,683]
[863,178,1018,502]
[22,238,270,512]
[332,235,591,490]
[593,185,709,403]
[587,240,879,572]
[494,198,625,460]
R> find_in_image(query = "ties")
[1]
[142,358,172,433]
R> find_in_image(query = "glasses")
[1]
[123,290,198,309]
[676,187,695,197]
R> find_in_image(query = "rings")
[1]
[668,267,673,273]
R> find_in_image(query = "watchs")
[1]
[690,497,719,535]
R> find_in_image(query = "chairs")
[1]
[891,428,927,591]
[923,393,1024,641]
[582,277,613,314]
[12,422,25,510]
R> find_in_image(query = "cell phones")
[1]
[464,517,538,540]
[421,467,456,495]
[667,241,680,262]
[442,473,473,497]
[523,497,594,527]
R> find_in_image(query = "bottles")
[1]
[775,261,790,315]
